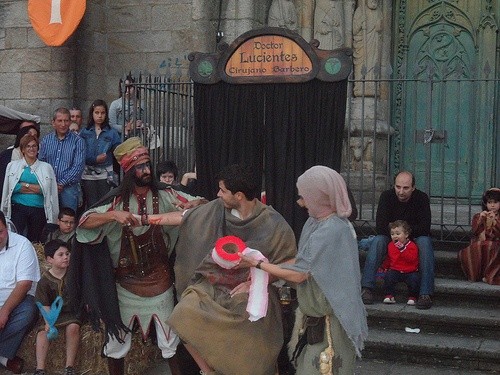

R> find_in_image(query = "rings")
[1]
[127,223,131,226]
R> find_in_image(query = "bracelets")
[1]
[141,213,148,227]
[255,259,264,270]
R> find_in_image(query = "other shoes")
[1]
[6,355,25,374]
[361,287,378,304]
[415,294,435,309]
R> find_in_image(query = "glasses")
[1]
[25,145,38,150]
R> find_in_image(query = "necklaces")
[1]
[96,128,101,131]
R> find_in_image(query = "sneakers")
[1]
[406,295,417,306]
[32,367,47,375]
[383,293,397,304]
[63,365,75,375]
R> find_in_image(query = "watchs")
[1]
[25,183,30,189]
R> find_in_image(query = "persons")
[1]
[457,186,500,285]
[108,75,157,182]
[6,120,40,149]
[229,165,368,375]
[1,133,60,243]
[360,169,435,309]
[80,98,123,211]
[155,160,178,184]
[170,161,197,191]
[69,106,87,208]
[38,107,86,217]
[376,219,420,306]
[33,238,83,375]
[0,125,41,204]
[127,167,297,375]
[75,135,210,375]
[0,208,42,375]
